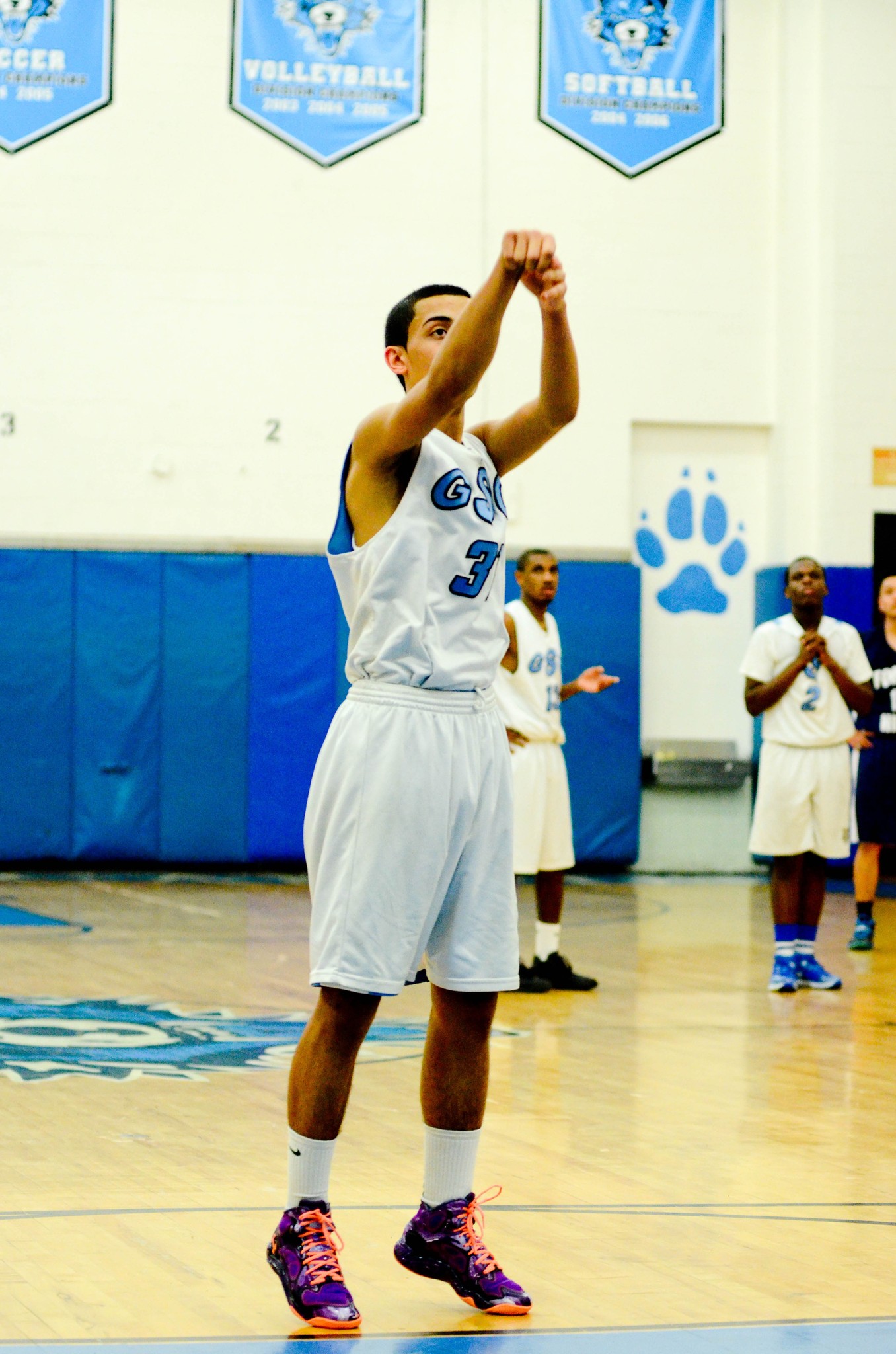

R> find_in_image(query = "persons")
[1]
[850,573,896,948]
[742,557,876,994]
[266,229,579,1330]
[496,549,620,993]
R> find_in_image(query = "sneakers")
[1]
[768,955,798,992]
[393,1186,533,1315]
[266,1199,362,1328]
[533,953,598,991]
[796,954,842,989]
[848,918,875,948]
[517,963,550,992]
